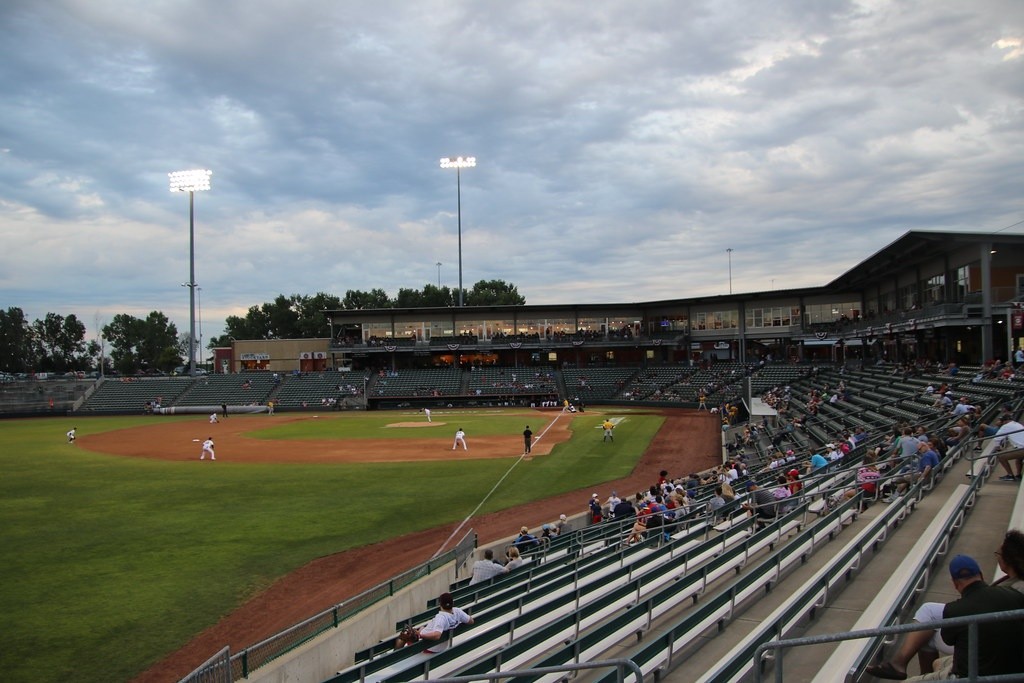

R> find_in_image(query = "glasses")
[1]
[919,445,926,451]
[994,551,1001,557]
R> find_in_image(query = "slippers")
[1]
[865,662,907,680]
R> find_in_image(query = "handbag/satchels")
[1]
[827,494,849,511]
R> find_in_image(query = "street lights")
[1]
[168,169,213,378]
[440,157,476,307]
[197,288,203,376]
[436,262,443,289]
[727,248,731,294]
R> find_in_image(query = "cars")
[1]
[0,372,17,381]
[139,369,165,376]
[86,372,100,377]
[77,371,88,376]
[170,366,188,376]
[195,368,207,375]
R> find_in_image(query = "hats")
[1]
[459,428,463,431]
[825,444,835,449]
[745,479,758,486]
[592,493,598,497]
[542,524,556,530]
[998,414,1013,420]
[521,526,528,532]
[526,425,529,428]
[788,469,798,476]
[440,593,454,609]
[74,428,77,430]
[641,503,649,509]
[949,555,981,579]
[958,397,966,402]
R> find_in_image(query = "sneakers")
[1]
[428,422,431,424]
[973,446,983,452]
[881,496,894,502]
[211,458,216,461]
[68,441,72,443]
[999,475,1022,481]
[452,448,456,451]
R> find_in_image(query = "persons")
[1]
[65,427,78,444]
[452,428,468,450]
[602,419,614,442]
[867,530,1024,679]
[200,437,216,461]
[900,554,1024,681]
[38,319,689,424]
[523,425,533,454]
[514,469,697,557]
[688,299,1024,531]
[393,592,475,651]
[469,545,523,586]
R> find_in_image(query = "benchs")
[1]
[82,302,1024,683]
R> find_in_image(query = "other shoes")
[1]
[622,540,630,546]
[525,453,527,454]
